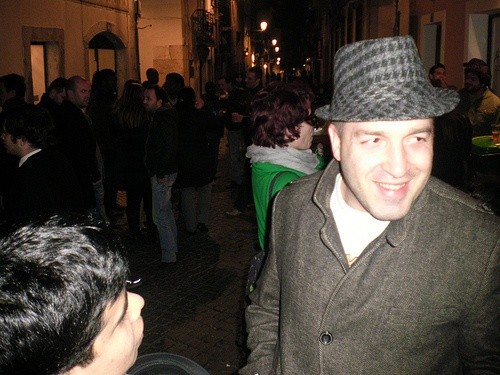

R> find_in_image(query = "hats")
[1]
[314,36,460,122]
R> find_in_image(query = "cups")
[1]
[313,128,331,144]
[491,124,500,140]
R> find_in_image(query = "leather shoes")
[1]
[126,277,146,290]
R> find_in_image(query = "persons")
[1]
[245,82,325,249]
[201,67,321,216]
[238,36,500,375]
[429,58,500,196]
[0,68,205,291]
[0,214,145,375]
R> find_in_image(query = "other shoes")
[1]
[198,223,208,233]
[157,262,175,272]
[225,209,242,216]
